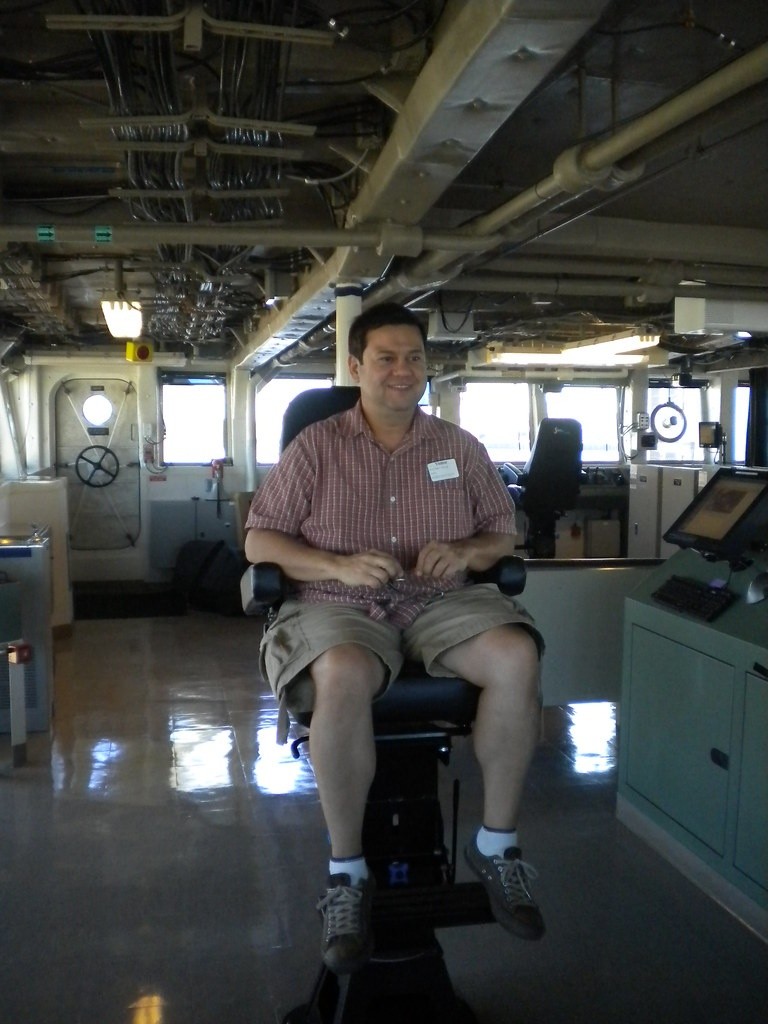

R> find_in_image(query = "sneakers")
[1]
[317,867,376,974]
[464,829,544,941]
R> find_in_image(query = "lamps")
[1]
[680,261,706,286]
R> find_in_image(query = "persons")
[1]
[243,303,546,974]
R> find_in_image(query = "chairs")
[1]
[502,416,585,508]
[240,388,527,726]
